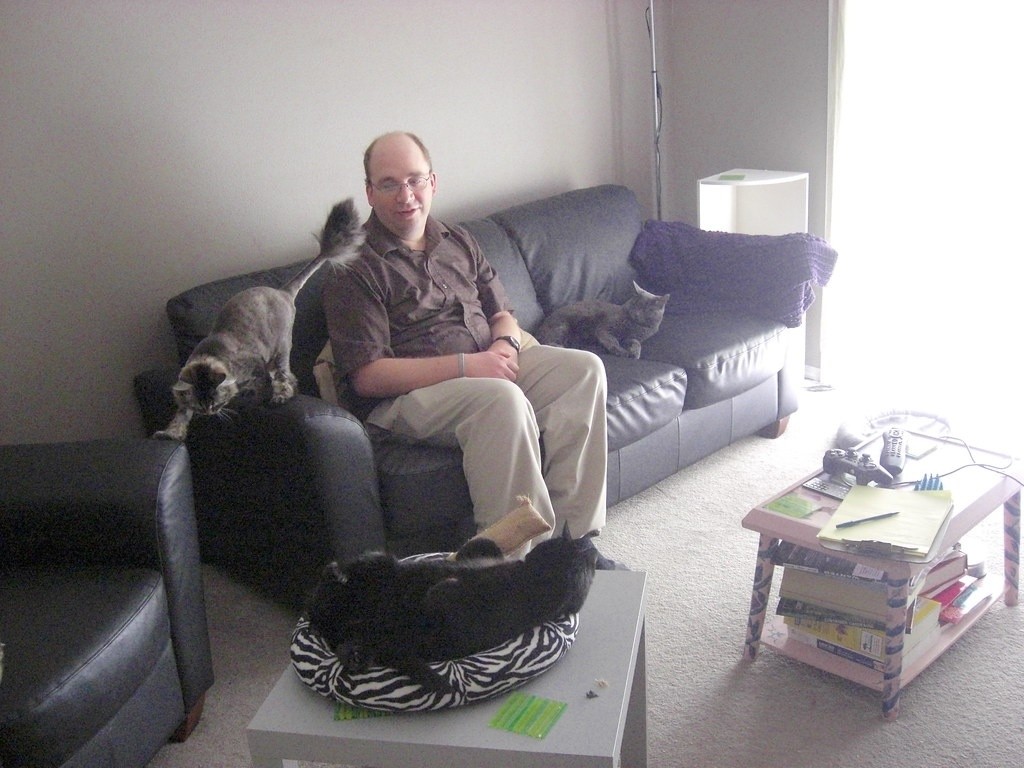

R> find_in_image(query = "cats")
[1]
[535,280,671,360]
[309,521,601,696]
[153,197,368,441]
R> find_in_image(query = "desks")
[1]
[741,426,1024,721]
[243,571,649,768]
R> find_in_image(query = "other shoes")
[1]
[562,519,630,570]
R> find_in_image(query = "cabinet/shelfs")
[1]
[698,170,808,236]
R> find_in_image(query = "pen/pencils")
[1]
[836,512,899,528]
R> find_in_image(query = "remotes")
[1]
[879,429,907,475]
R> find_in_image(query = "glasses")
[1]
[364,178,431,194]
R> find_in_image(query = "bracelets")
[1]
[458,353,464,377]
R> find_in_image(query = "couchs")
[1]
[0,439,214,768]
[133,182,838,616]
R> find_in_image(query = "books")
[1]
[905,435,937,459]
[768,540,994,676]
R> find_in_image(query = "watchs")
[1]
[492,336,520,354]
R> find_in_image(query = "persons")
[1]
[325,131,635,571]
